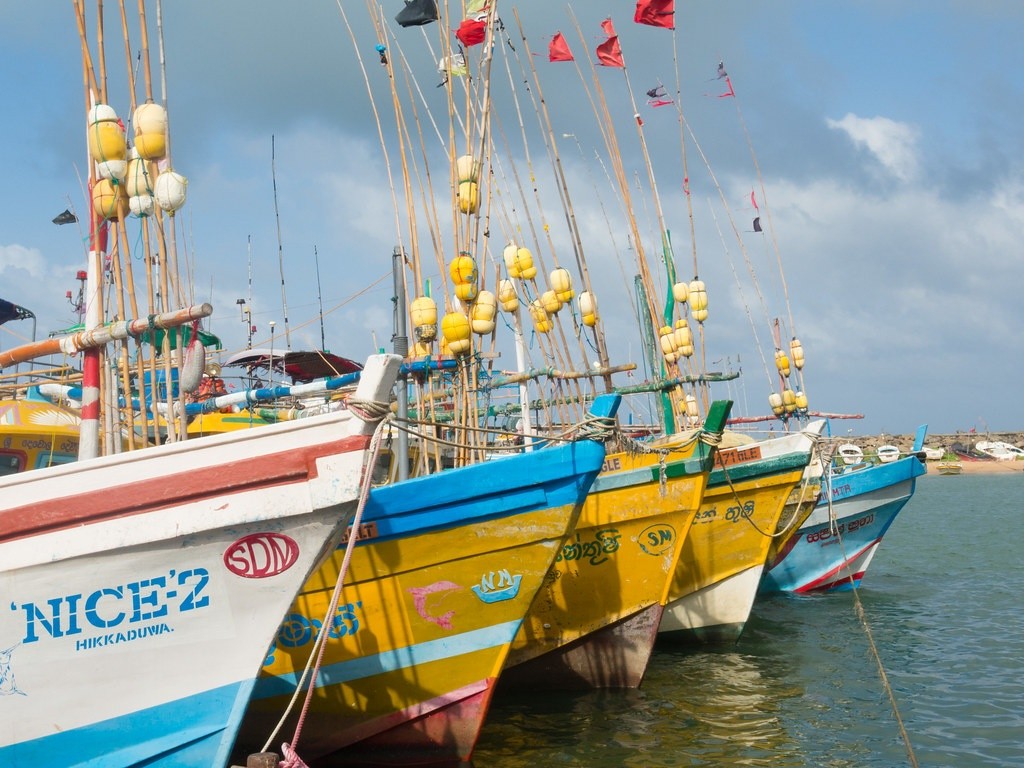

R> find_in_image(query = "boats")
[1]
[976,440,1017,461]
[0,1,401,768]
[121,1,925,693]
[0,1,622,768]
[911,446,946,461]
[876,444,900,462]
[955,449,995,462]
[994,441,1024,457]
[839,443,865,466]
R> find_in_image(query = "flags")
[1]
[597,37,623,73]
[633,1,674,28]
